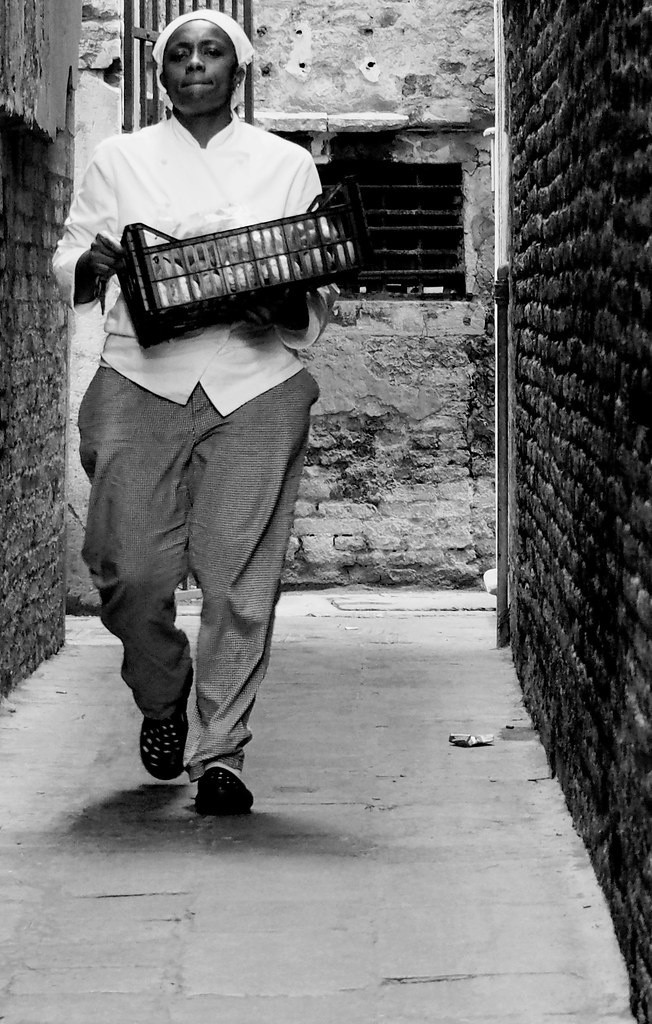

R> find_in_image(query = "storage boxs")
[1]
[106,170,377,351]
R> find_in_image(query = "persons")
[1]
[50,11,345,813]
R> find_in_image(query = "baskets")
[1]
[111,172,364,350]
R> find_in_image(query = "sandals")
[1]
[192,759,253,815]
[143,668,193,780]
